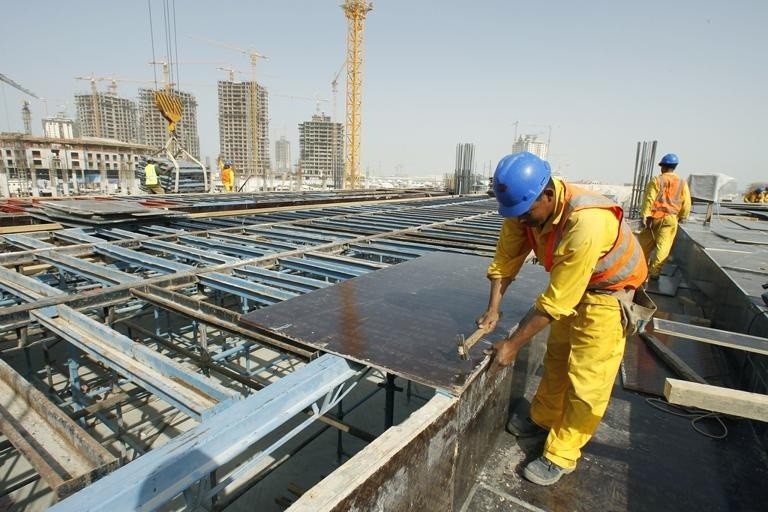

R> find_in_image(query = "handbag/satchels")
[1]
[613,283,658,337]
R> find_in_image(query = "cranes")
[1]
[0,74,47,104]
[331,61,346,123]
[273,91,330,118]
[149,57,192,96]
[201,37,270,176]
[214,62,252,82]
[104,76,170,96]
[74,73,138,138]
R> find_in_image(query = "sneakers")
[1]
[507,416,548,437]
[523,456,578,486]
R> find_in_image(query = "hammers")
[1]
[456,311,503,360]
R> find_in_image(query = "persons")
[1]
[474,151,651,488]
[144,155,173,195]
[217,156,235,194]
[744,186,768,203]
[634,154,692,281]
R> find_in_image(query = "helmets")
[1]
[657,154,678,165]
[493,152,551,217]
